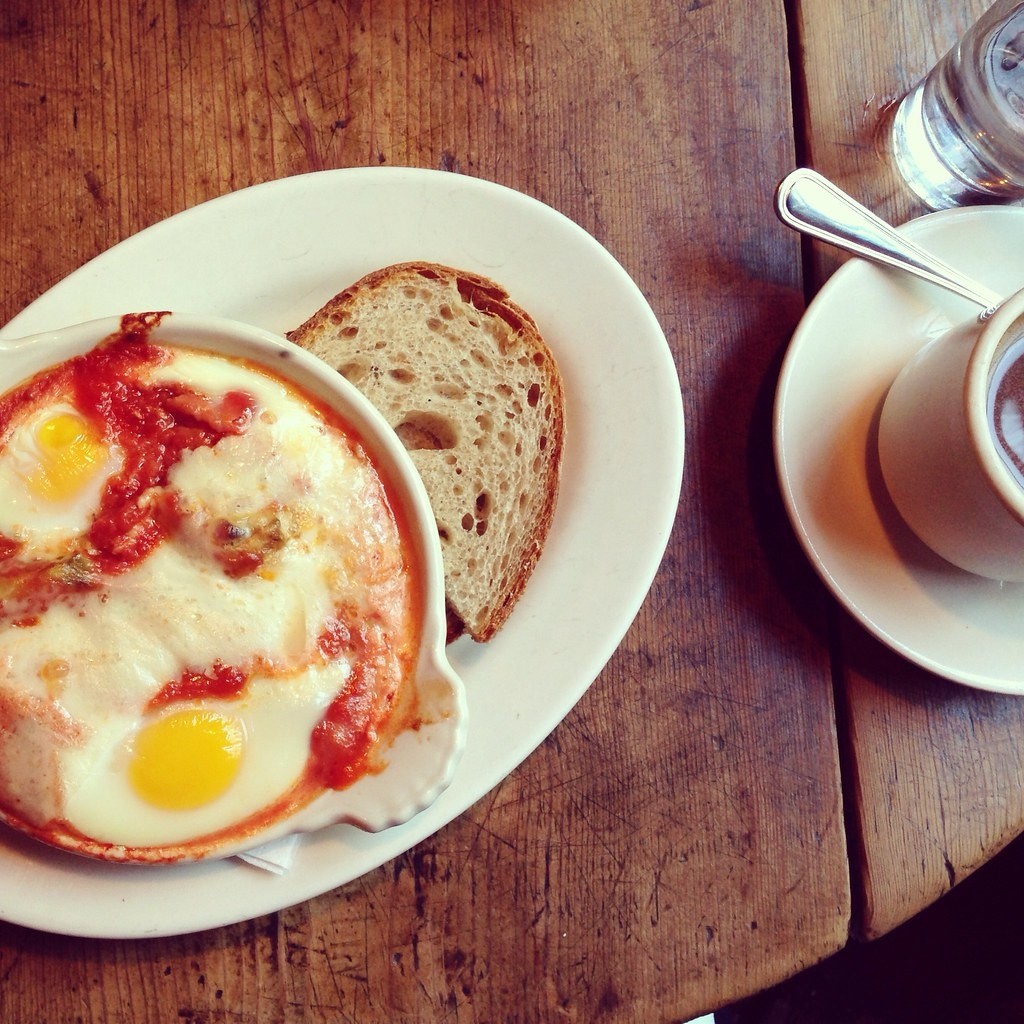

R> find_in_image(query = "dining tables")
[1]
[0,0,1024,1024]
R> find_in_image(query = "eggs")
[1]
[56,655,352,851]
[0,390,131,546]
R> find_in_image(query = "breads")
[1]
[287,259,565,643]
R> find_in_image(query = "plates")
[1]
[772,204,1024,696]
[0,168,685,939]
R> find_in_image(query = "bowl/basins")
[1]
[0,312,467,867]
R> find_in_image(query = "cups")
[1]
[888,0,1024,211]
[876,287,1024,584]
